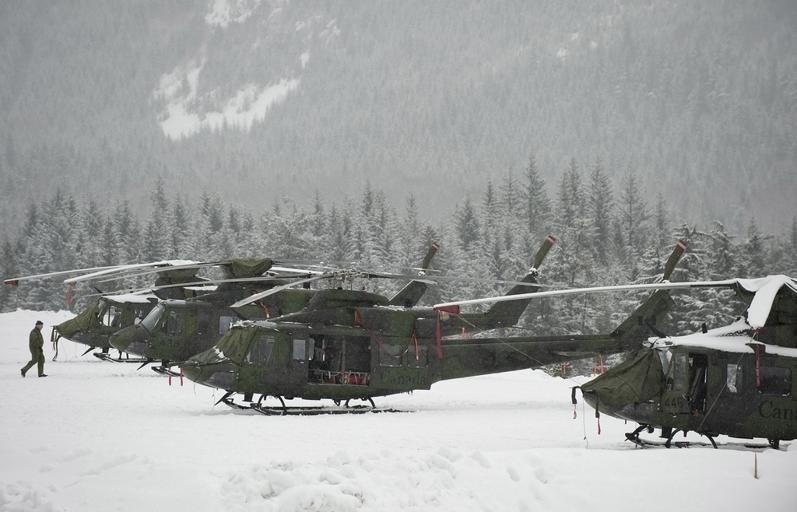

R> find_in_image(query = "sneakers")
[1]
[38,374,47,377]
[21,368,25,377]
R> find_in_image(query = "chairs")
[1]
[307,346,329,381]
[687,366,706,412]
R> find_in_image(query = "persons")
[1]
[20,319,47,378]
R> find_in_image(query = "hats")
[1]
[36,320,43,325]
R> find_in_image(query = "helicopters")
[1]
[433,273,797,451]
